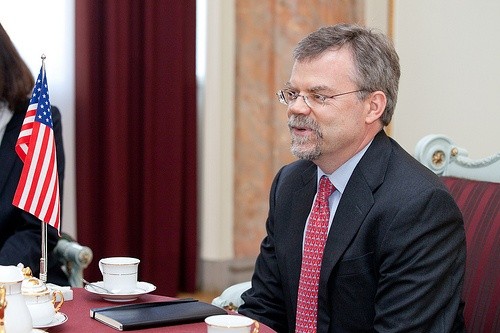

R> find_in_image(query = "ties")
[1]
[294,174,338,333]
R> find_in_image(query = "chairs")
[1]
[210,133,500,333]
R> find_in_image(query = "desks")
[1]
[0,288,279,333]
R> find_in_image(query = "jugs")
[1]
[0,279,33,333]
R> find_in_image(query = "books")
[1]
[90,298,229,331]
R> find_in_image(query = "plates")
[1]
[85,280,156,302]
[36,311,68,330]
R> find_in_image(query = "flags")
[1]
[12,66,62,238]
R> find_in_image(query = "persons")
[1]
[0,22,73,286]
[237,23,467,333]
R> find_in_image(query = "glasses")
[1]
[276,86,368,109]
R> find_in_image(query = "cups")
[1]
[99,257,141,293]
[205,313,259,333]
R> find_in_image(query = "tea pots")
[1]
[21,276,64,326]
